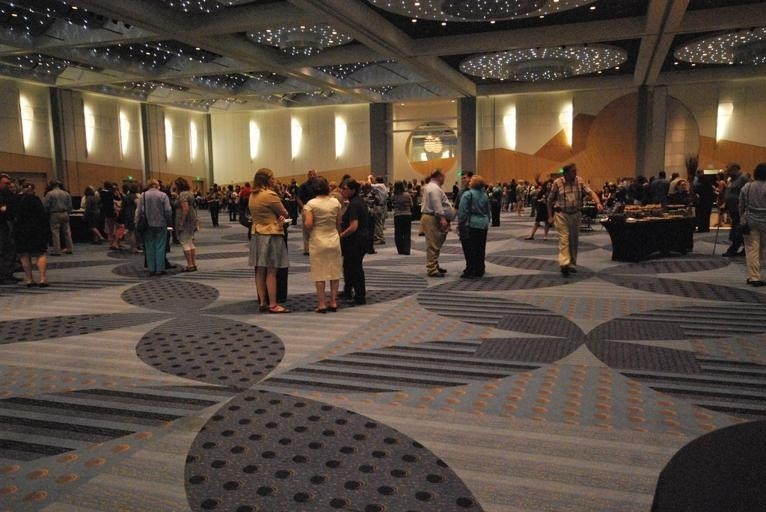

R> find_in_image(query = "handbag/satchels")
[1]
[459,227,472,242]
[136,213,149,234]
[734,224,750,235]
[193,215,201,233]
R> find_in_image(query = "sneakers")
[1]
[560,264,578,274]
[374,238,385,245]
[746,278,760,287]
[346,298,366,305]
[337,292,352,299]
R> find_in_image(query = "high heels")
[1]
[314,305,337,313]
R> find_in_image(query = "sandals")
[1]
[258,303,267,313]
[267,305,291,313]
[179,265,197,271]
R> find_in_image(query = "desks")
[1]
[599,215,698,263]
[68,212,87,243]
[581,206,598,232]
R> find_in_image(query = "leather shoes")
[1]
[26,281,36,288]
[39,280,50,288]
[428,269,446,278]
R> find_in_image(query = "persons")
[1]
[738,161,766,288]
[247,168,294,314]
[0,174,24,285]
[270,169,349,256]
[337,177,376,309]
[248,179,299,306]
[362,161,755,279]
[11,182,54,289]
[12,176,251,280]
[302,174,343,313]
